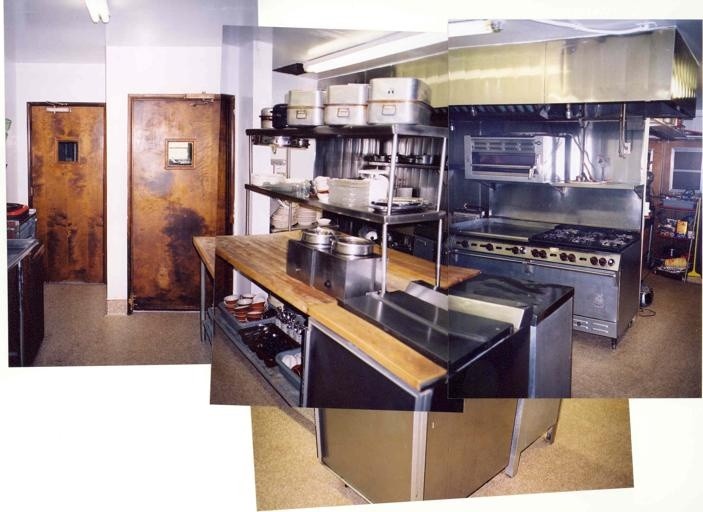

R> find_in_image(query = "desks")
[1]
[193,236,216,342]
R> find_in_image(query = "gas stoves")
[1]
[527,222,638,269]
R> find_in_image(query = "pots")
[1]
[259,104,286,129]
[372,152,448,165]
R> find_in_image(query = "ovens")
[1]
[464,132,566,183]
[446,235,619,340]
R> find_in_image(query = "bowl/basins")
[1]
[223,294,265,321]
[282,353,302,376]
[317,218,331,224]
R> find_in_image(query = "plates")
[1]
[272,208,318,227]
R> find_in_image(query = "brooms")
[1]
[687,198,702,283]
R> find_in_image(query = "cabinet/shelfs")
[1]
[246,126,450,295]
[646,206,698,284]
[8,245,45,366]
